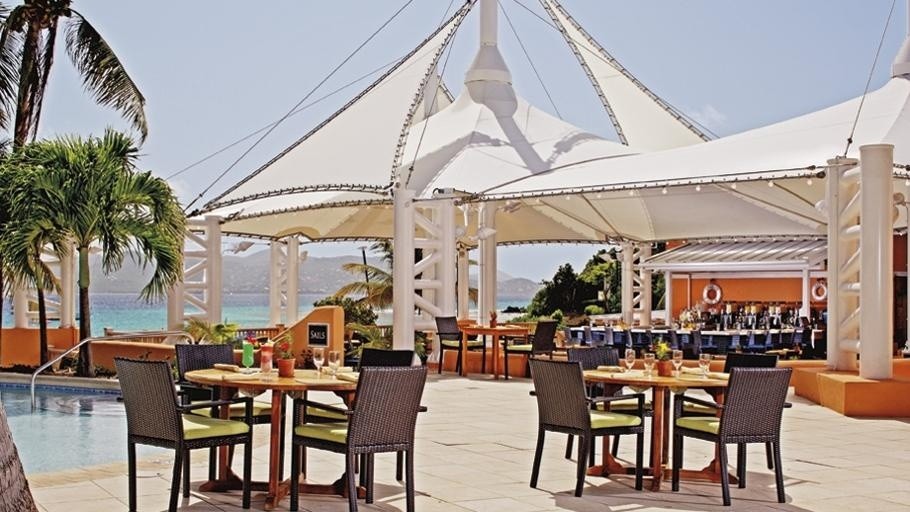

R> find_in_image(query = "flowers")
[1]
[275,333,293,359]
[652,336,673,361]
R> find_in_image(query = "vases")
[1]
[277,358,296,377]
[656,360,674,377]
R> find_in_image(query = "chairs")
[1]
[528,356,644,497]
[566,347,653,468]
[290,366,428,512]
[435,299,828,382]
[113,356,253,511]
[303,348,415,485]
[680,354,780,477]
[106,332,429,511]
[175,344,273,483]
[672,366,793,506]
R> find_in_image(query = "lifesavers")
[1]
[702,285,721,306]
[812,283,827,301]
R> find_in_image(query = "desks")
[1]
[579,367,739,492]
[184,366,368,511]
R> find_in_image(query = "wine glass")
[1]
[643,353,658,381]
[259,344,275,384]
[327,351,342,381]
[242,339,253,374]
[672,349,684,380]
[313,346,326,376]
[697,353,712,381]
[624,348,637,377]
